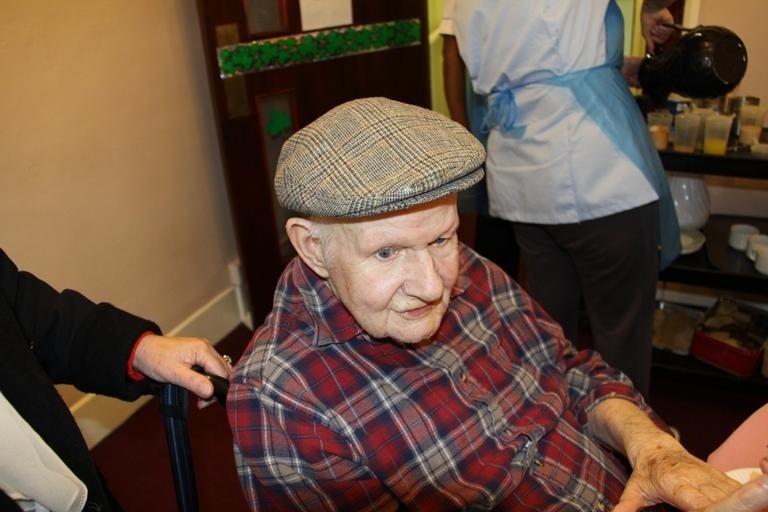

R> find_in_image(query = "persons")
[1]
[439,0,674,403]
[0,247,232,512]
[226,96,768,512]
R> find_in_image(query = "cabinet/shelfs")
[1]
[652,128,768,399]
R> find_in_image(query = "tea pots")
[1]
[636,25,748,101]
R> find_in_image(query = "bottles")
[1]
[738,95,760,145]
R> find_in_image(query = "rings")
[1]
[223,354,232,364]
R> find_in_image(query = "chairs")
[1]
[158,363,231,512]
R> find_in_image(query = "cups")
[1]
[730,223,768,275]
[647,111,733,155]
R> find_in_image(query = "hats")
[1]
[274,96,487,219]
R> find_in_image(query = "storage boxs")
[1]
[690,296,768,377]
